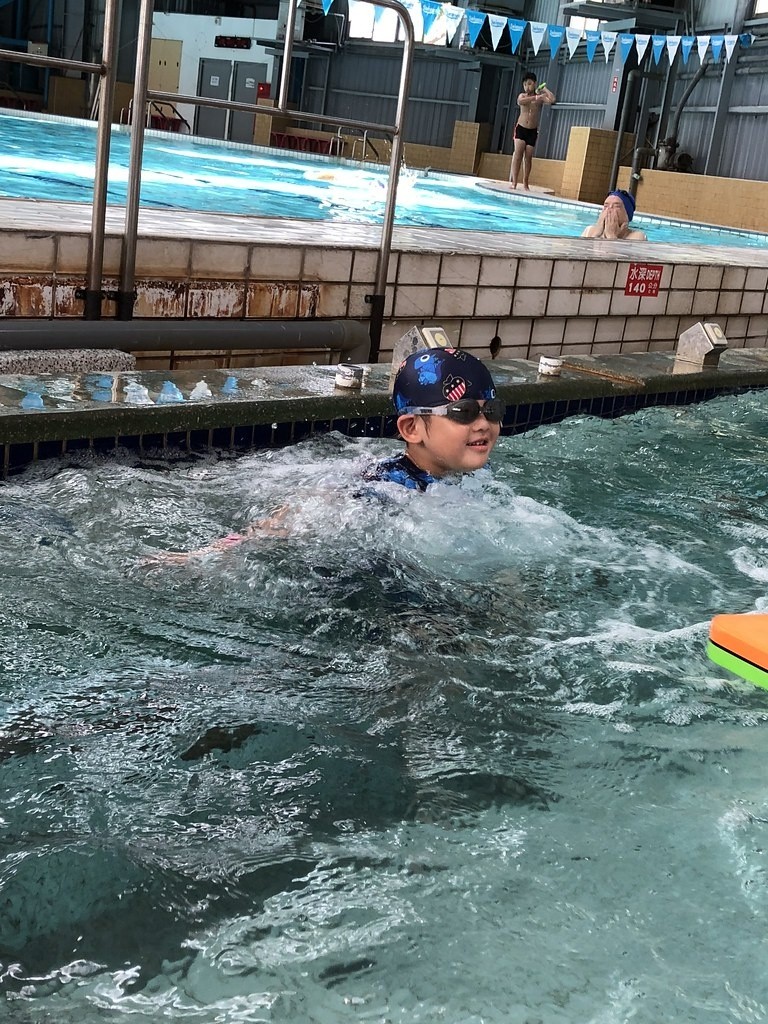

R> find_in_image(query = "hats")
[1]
[607,187,636,222]
[392,347,496,411]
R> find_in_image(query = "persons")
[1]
[580,188,647,241]
[365,345,505,494]
[509,72,556,191]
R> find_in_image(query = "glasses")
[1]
[400,398,505,424]
[607,190,629,198]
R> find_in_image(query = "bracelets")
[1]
[536,95,537,100]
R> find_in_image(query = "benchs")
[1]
[272,131,349,156]
[118,109,187,131]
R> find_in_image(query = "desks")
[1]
[48,75,134,123]
